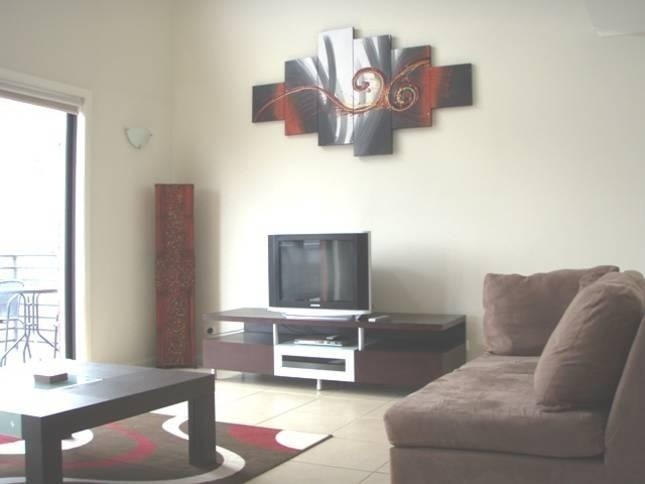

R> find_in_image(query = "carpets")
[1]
[0,412,333,483]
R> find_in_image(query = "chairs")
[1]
[1,280,24,366]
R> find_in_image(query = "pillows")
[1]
[480,263,644,413]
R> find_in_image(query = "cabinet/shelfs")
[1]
[198,308,468,393]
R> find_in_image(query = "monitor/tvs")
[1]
[267,229,372,320]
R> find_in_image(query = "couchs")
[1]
[383,286,644,483]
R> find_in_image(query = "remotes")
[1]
[326,332,343,340]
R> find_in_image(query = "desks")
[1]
[0,287,59,368]
